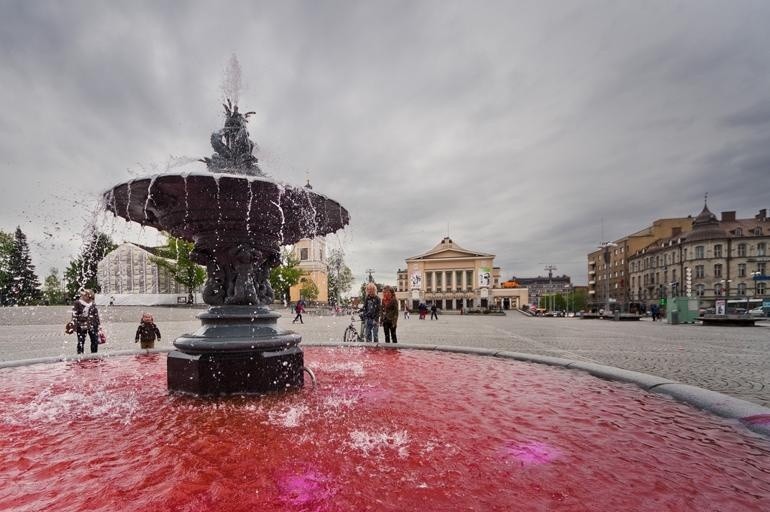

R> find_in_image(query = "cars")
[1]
[751,306,770,317]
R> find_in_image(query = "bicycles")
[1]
[344,308,367,343]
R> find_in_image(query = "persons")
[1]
[355,283,399,343]
[430,303,438,320]
[292,300,305,324]
[134,313,161,349]
[650,304,661,322]
[418,302,428,319]
[403,304,411,319]
[65,289,102,354]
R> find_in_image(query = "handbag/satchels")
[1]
[97,333,106,344]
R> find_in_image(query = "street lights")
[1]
[544,264,557,312]
[365,269,375,282]
[597,240,618,313]
[750,271,761,298]
[721,278,732,296]
